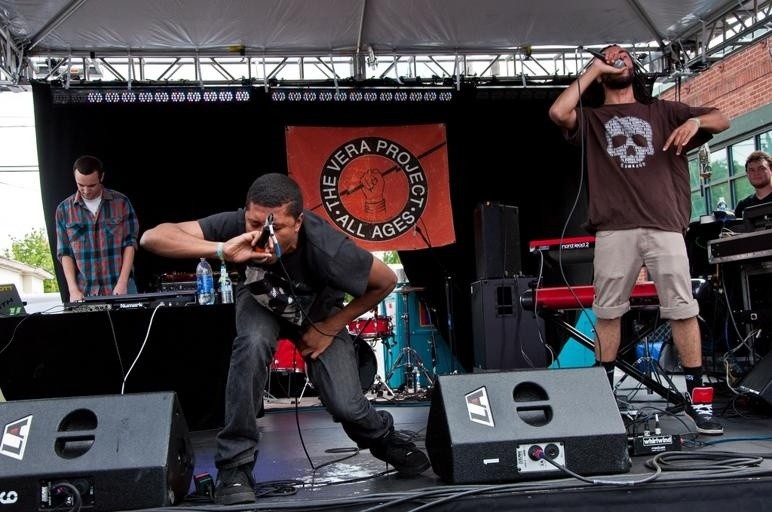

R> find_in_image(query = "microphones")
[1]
[580,46,624,68]
[252,213,274,264]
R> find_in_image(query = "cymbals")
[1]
[391,284,424,294]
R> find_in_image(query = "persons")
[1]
[54,156,140,304]
[735,150,772,218]
[137,173,431,506]
[548,44,732,435]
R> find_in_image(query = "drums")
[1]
[268,338,305,374]
[307,334,377,399]
[349,315,394,339]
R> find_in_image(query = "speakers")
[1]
[426,367,629,484]
[739,348,772,405]
[0,392,196,512]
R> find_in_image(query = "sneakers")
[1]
[211,463,257,504]
[683,387,725,434]
[369,429,431,476]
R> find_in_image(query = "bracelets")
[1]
[688,118,700,127]
[216,242,224,261]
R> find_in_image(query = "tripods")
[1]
[380,295,434,393]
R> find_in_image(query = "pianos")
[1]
[65,289,198,305]
[528,236,595,262]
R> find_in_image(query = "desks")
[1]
[0,300,265,431]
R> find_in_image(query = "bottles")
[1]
[219,264,234,304]
[196,256,215,306]
[411,366,420,391]
[717,196,727,210]
